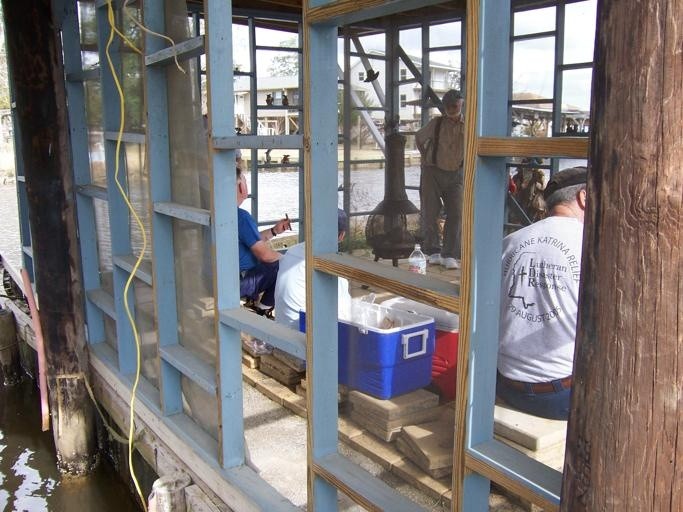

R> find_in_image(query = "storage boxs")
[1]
[302,296,435,400]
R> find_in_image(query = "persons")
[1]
[415,90,465,269]
[275,208,353,329]
[236,167,292,320]
[496,166,587,420]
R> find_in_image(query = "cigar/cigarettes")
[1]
[285,212,292,231]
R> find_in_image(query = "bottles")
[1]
[407,244,426,275]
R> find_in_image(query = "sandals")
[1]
[251,303,275,320]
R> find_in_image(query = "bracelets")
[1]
[270,227,277,236]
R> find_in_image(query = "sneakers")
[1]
[427,253,459,269]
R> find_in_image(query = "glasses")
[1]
[444,104,458,109]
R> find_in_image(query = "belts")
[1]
[501,376,574,394]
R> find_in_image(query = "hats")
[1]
[442,88,465,103]
[543,166,588,199]
[338,207,348,233]
